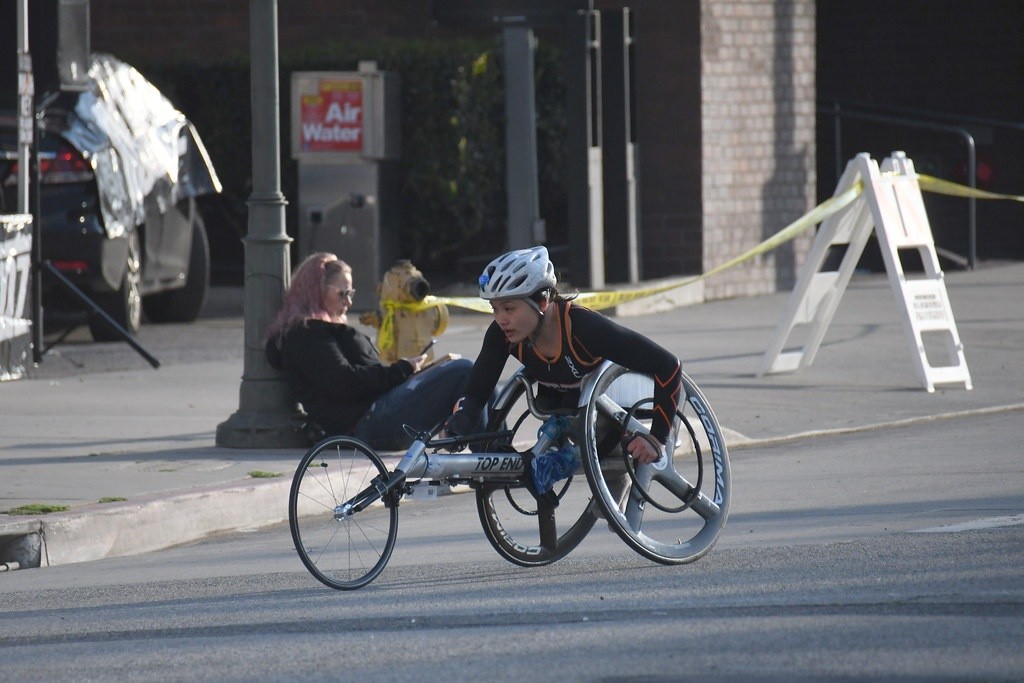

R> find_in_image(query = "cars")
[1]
[1,55,211,342]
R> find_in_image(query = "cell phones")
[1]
[418,339,437,356]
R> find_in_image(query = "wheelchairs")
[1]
[287,363,733,592]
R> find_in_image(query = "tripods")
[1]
[27,93,163,367]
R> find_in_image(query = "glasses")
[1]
[324,283,356,300]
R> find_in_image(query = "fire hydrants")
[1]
[359,259,450,373]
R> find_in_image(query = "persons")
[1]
[268,252,533,488]
[441,247,681,523]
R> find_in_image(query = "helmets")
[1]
[478,246,558,299]
